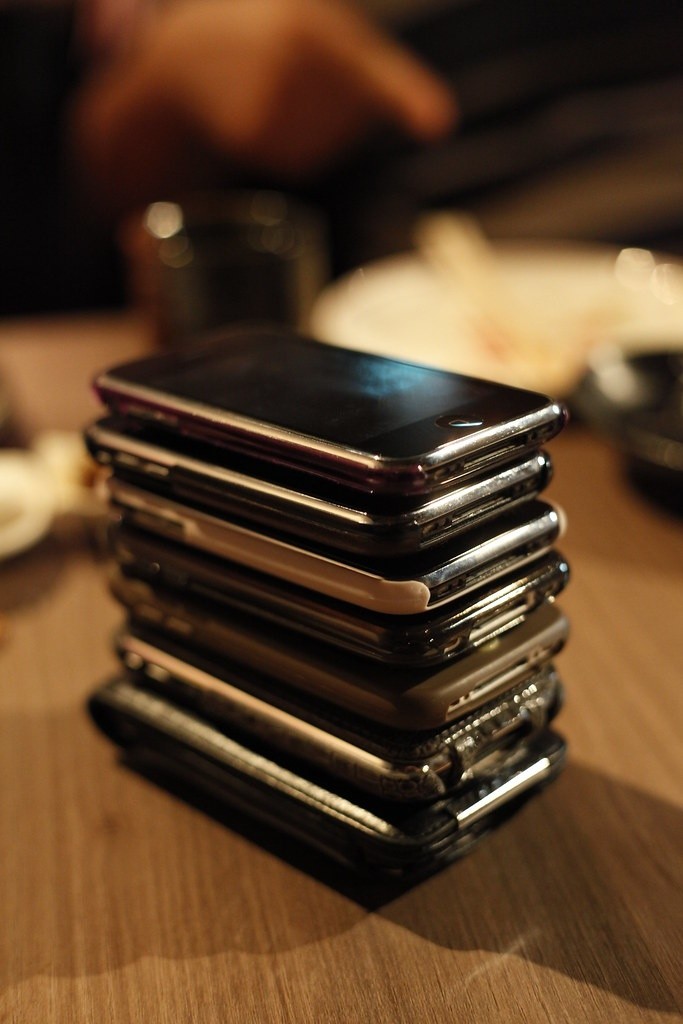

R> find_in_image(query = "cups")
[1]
[141,204,315,351]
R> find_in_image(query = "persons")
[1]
[70,1,460,189]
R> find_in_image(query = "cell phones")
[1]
[83,315,571,905]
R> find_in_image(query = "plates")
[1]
[0,455,51,556]
[316,244,683,399]
